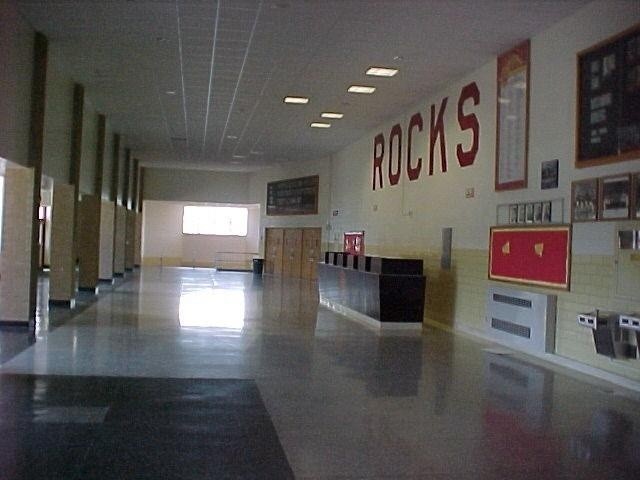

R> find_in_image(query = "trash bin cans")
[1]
[252,258,265,274]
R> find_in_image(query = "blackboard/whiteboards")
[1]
[266,174,319,217]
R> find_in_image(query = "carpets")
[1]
[0,372,295,480]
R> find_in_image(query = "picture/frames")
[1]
[509,200,552,225]
[570,171,640,223]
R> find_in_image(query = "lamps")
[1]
[577,309,640,361]
